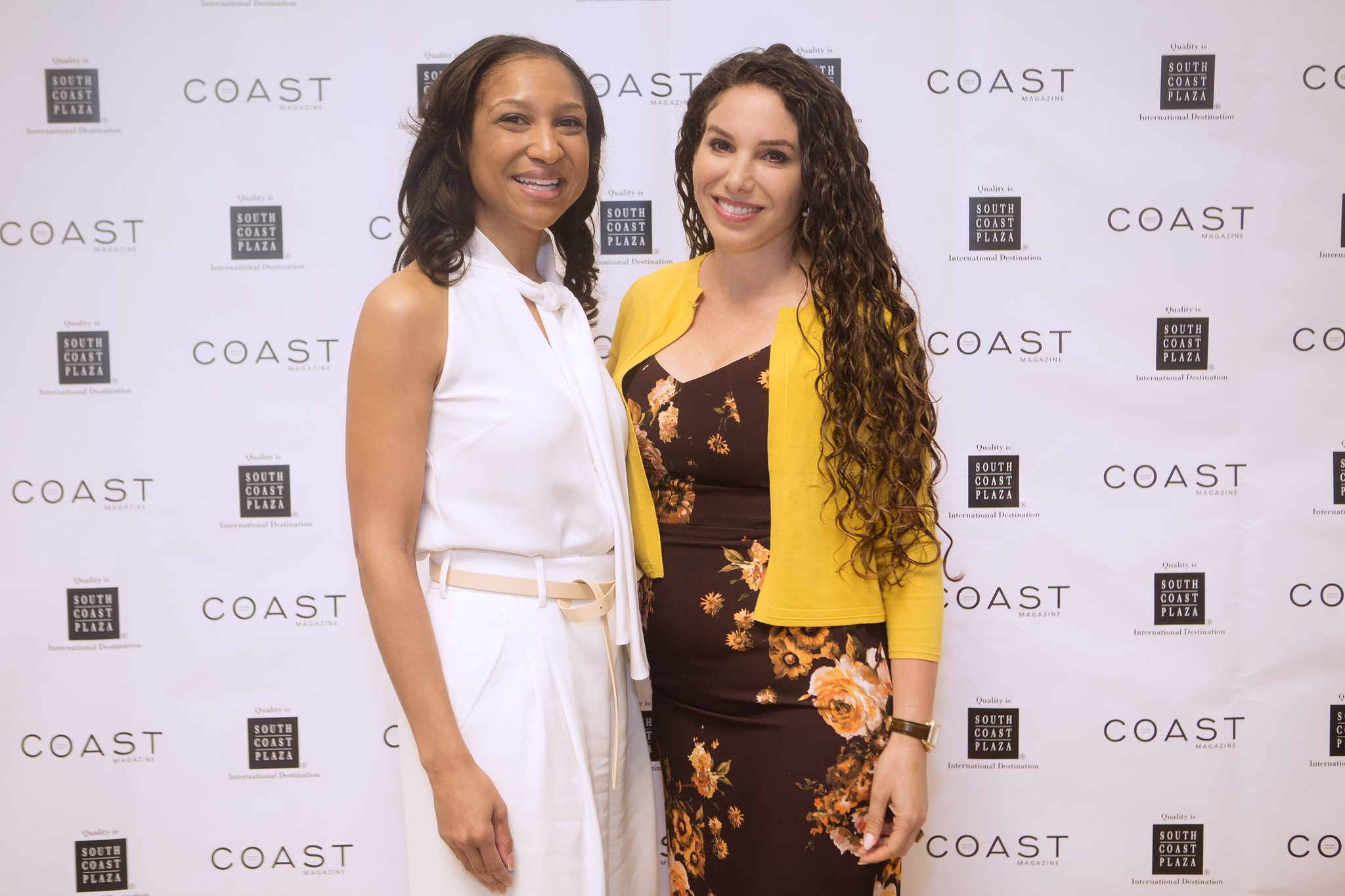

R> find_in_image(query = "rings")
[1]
[915,829,926,844]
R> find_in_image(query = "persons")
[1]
[345,32,652,896]
[605,43,966,896]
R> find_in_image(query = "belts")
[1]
[415,555,620,788]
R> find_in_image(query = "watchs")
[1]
[884,715,941,752]
